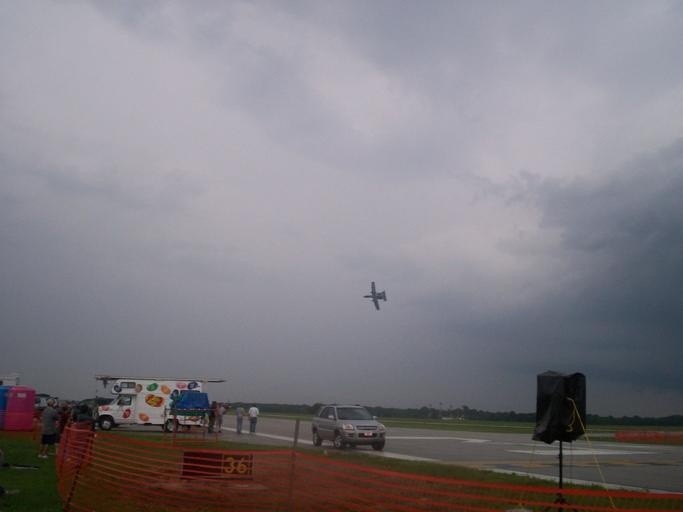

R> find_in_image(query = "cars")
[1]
[71,398,113,422]
[311,405,386,450]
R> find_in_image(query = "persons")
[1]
[249,404,259,434]
[237,405,246,434]
[207,401,217,432]
[215,403,227,434]
[61,404,68,436]
[37,398,59,459]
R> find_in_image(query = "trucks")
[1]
[95,379,202,433]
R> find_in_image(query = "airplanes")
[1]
[363,281,386,310]
